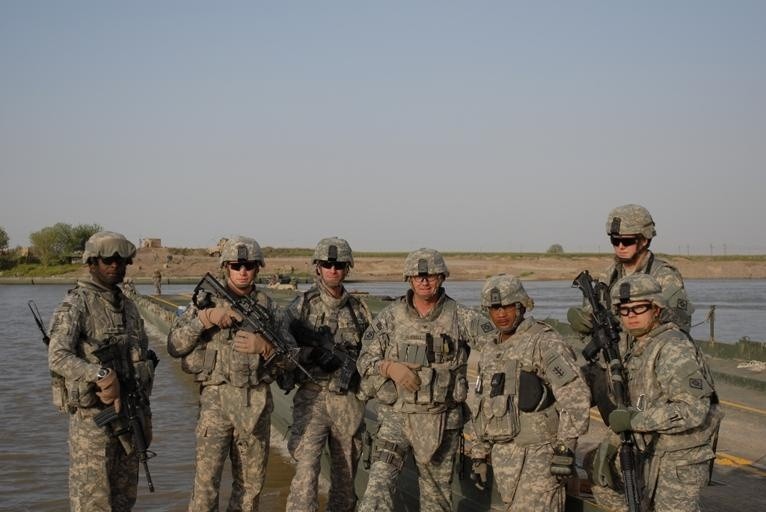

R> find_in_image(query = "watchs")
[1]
[96,367,110,379]
[551,444,575,457]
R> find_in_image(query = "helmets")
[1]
[83,231,135,265]
[403,248,449,282]
[481,276,534,311]
[313,238,354,269]
[605,204,656,239]
[220,237,265,268]
[610,274,666,316]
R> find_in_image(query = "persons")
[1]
[291,276,298,289]
[129,279,142,295]
[354,247,464,512]
[270,238,369,512]
[41,230,157,512]
[123,278,136,294]
[152,268,162,295]
[595,203,697,335]
[464,276,593,512]
[165,237,279,512]
[592,269,725,512]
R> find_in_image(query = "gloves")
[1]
[231,331,274,358]
[470,458,487,491]
[567,307,593,333]
[96,368,121,414]
[378,361,421,392]
[550,443,575,481]
[198,309,242,330]
[609,408,639,431]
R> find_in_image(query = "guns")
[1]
[592,358,640,512]
[191,273,319,389]
[93,343,155,492]
[288,321,361,390]
[568,269,624,413]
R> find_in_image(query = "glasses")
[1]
[618,304,652,316]
[320,261,347,269]
[230,262,257,270]
[610,235,639,246]
[101,257,132,266]
